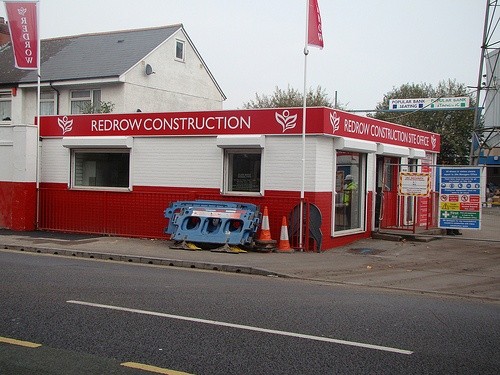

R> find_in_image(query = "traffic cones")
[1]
[274,215,295,253]
[253,205,278,253]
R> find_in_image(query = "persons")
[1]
[342,175,359,228]
[446,229,462,236]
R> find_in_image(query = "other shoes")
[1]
[446,232,455,235]
[455,232,462,235]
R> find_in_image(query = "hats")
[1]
[345,175,352,180]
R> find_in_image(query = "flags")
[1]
[306,0,323,49]
[4,0,38,70]
